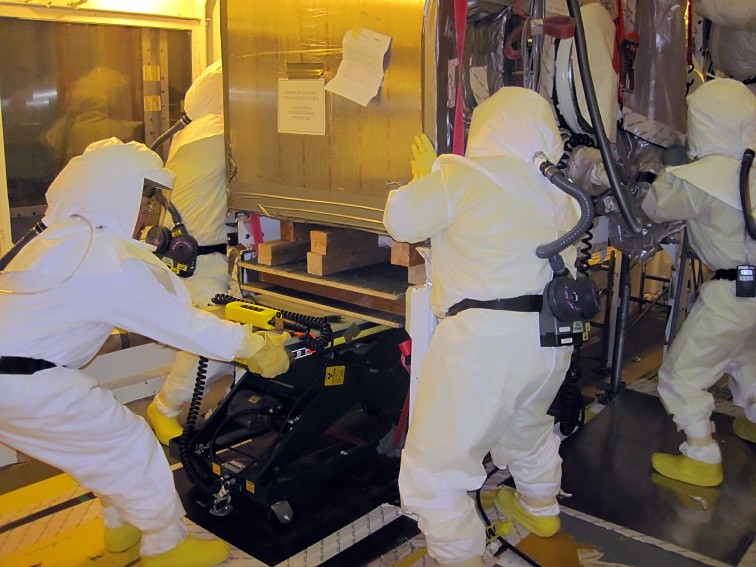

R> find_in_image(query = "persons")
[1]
[0,137,291,565]
[381,87,583,567]
[642,78,756,488]
[145,60,228,445]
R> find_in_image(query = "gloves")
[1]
[245,329,291,378]
[409,133,438,181]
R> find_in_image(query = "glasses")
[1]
[144,185,156,199]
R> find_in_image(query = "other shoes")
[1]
[733,414,756,442]
[147,401,184,446]
[103,522,143,553]
[651,473,721,511]
[141,534,230,567]
[490,485,560,537]
[651,451,722,487]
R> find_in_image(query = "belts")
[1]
[0,355,67,375]
[197,243,227,254]
[445,294,543,312]
